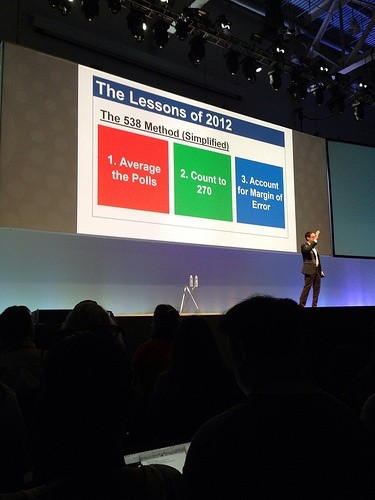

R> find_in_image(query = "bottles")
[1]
[194,274,199,287]
[189,274,193,287]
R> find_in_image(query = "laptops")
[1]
[123,442,191,474]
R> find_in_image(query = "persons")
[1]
[299,230,324,307]
[0,296,375,500]
